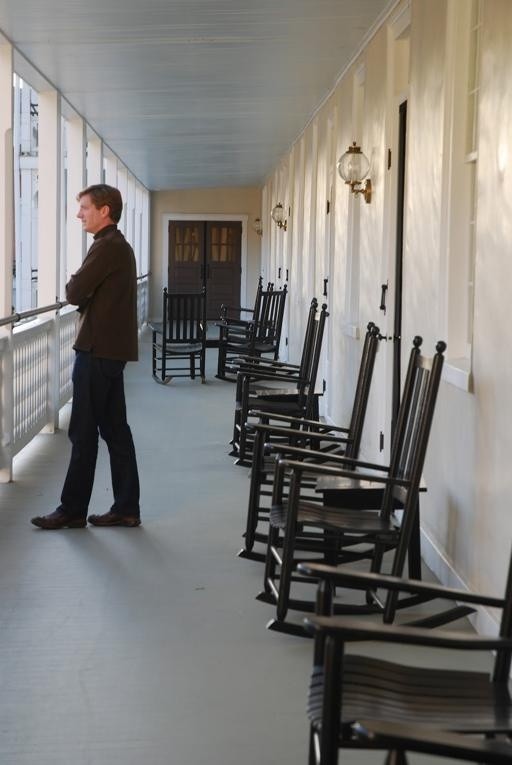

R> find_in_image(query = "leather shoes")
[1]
[31,510,88,529]
[88,511,141,527]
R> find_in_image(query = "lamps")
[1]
[337,141,371,203]
[252,217,263,235]
[271,202,287,231]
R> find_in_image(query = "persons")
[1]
[29,184,141,528]
